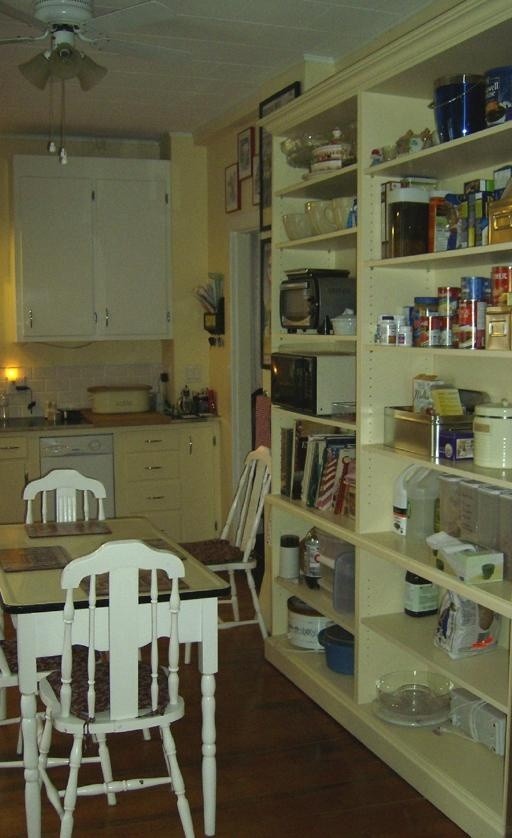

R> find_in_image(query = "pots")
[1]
[58,408,81,421]
[87,384,156,414]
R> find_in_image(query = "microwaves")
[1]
[278,276,356,334]
[269,351,355,416]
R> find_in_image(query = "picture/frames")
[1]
[221,162,244,213]
[236,125,255,181]
[255,80,302,229]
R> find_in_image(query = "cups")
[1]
[321,195,354,229]
[382,129,437,162]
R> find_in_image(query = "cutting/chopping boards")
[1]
[80,407,171,428]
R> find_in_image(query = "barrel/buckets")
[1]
[406,468,440,542]
[424,72,487,145]
[390,463,422,537]
[481,64,512,130]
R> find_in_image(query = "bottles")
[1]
[48,401,57,421]
[471,398,511,472]
[305,528,324,589]
[279,534,300,581]
[376,314,413,346]
[177,385,218,418]
[0,391,11,421]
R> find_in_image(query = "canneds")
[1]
[413,267,512,347]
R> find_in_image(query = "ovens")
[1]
[39,433,115,523]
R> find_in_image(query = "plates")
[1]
[370,699,453,729]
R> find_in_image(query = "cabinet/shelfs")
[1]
[0,433,39,523]
[115,420,222,540]
[261,0,512,834]
[8,152,94,337]
[95,156,174,339]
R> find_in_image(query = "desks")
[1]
[1,508,230,836]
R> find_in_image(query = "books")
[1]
[281,418,357,521]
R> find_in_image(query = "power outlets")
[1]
[8,375,26,394]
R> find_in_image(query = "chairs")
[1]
[37,533,200,835]
[179,439,272,637]
[1,628,100,770]
[21,466,107,523]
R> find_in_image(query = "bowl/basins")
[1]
[283,213,312,240]
[327,308,357,334]
[317,624,356,676]
[306,201,335,235]
[376,669,454,716]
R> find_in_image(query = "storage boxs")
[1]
[382,405,474,458]
[315,527,355,585]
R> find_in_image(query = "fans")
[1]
[0,0,173,90]
